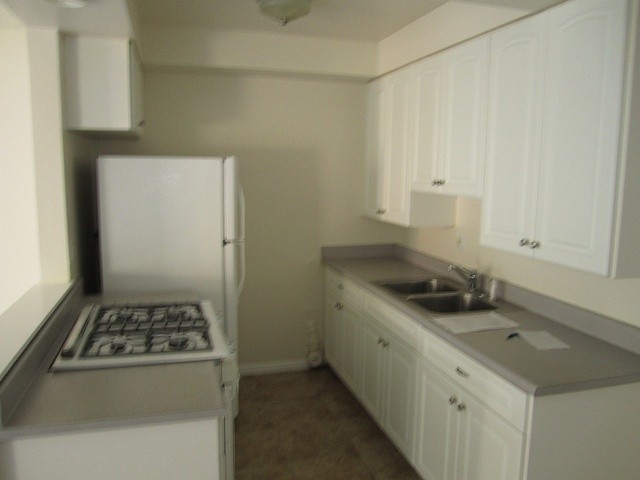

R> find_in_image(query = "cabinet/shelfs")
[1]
[415,328,640,480]
[478,0,640,279]
[356,67,456,229]
[361,292,424,468]
[63,35,146,138]
[412,36,491,198]
[323,266,366,402]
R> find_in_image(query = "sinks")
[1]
[408,293,497,313]
[383,277,460,294]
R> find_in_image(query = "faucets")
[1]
[447,263,478,294]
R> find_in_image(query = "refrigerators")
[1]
[95,153,247,418]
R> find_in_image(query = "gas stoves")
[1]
[49,298,228,371]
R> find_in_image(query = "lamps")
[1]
[259,0,312,26]
